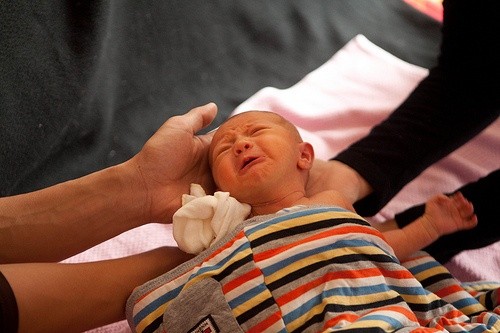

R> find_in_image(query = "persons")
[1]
[305,1,500,265]
[1,103,221,333]
[210,111,477,264]
